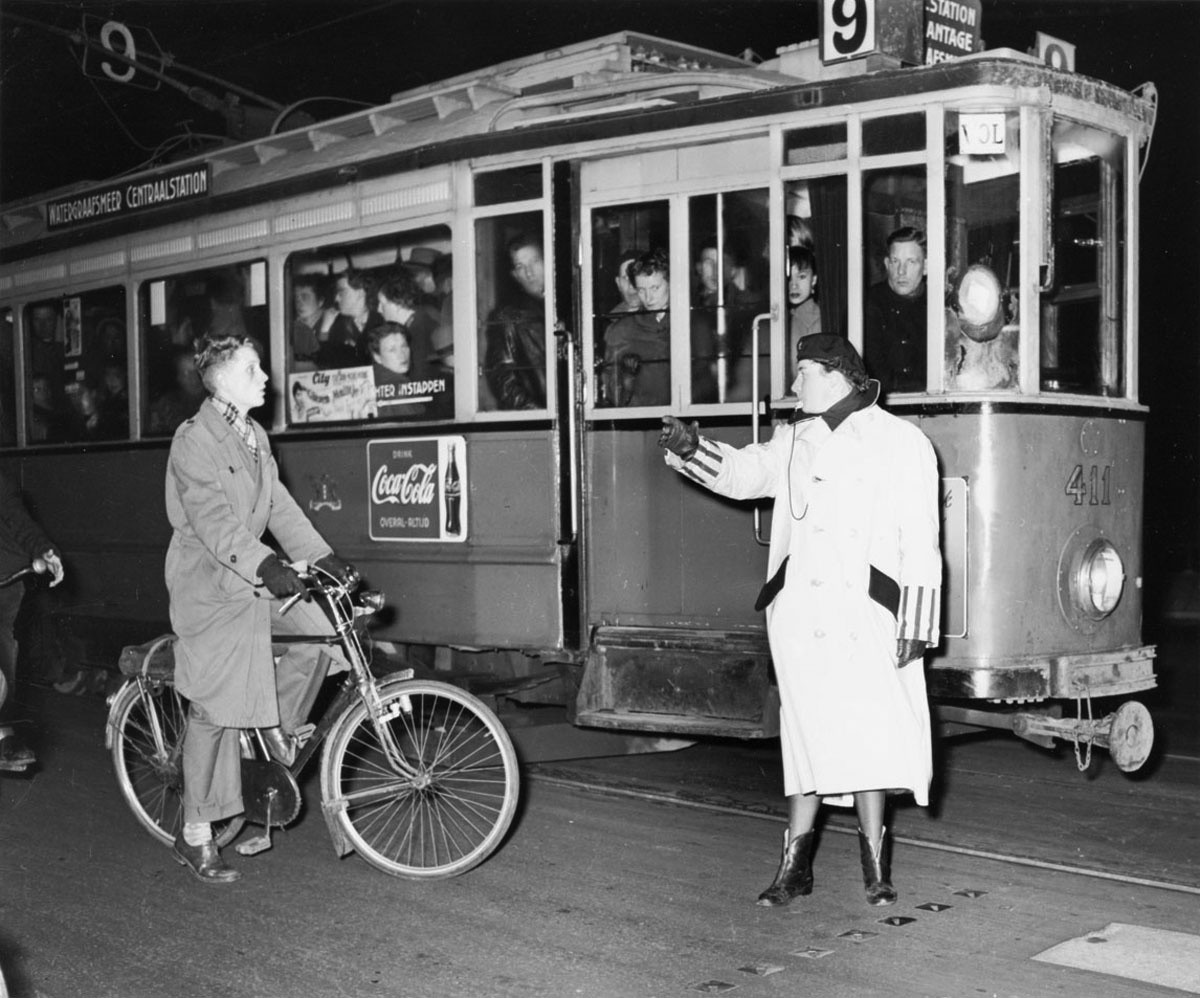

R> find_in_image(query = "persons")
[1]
[30,225,1020,447]
[656,337,943,906]
[164,336,356,883]
[0,480,65,770]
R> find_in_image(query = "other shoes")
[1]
[169,831,243,883]
[0,734,37,765]
[262,726,294,768]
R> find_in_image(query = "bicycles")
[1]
[105,558,521,882]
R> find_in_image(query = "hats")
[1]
[428,325,454,362]
[403,248,442,271]
[796,332,868,388]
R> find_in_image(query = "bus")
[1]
[0,0,1163,783]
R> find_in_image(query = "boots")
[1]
[857,826,898,907]
[755,827,821,907]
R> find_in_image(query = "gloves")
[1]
[255,554,311,603]
[895,639,927,668]
[315,554,357,585]
[658,416,700,459]
